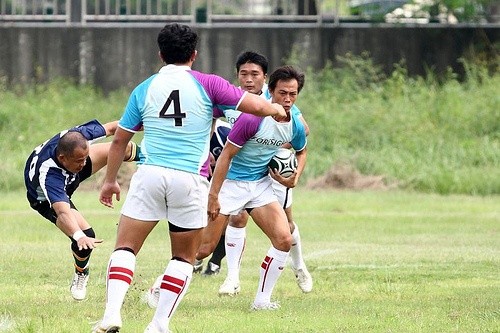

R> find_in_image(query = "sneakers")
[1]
[292,263,313,293]
[252,302,282,310]
[70,269,89,300]
[218,278,241,294]
[146,274,163,309]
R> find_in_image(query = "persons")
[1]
[147,52,312,310]
[24,119,141,302]
[93,24,287,333]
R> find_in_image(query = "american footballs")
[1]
[270,147,298,178]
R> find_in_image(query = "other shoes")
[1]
[88,318,122,333]
[192,260,203,273]
[144,323,172,333]
[202,262,220,277]
